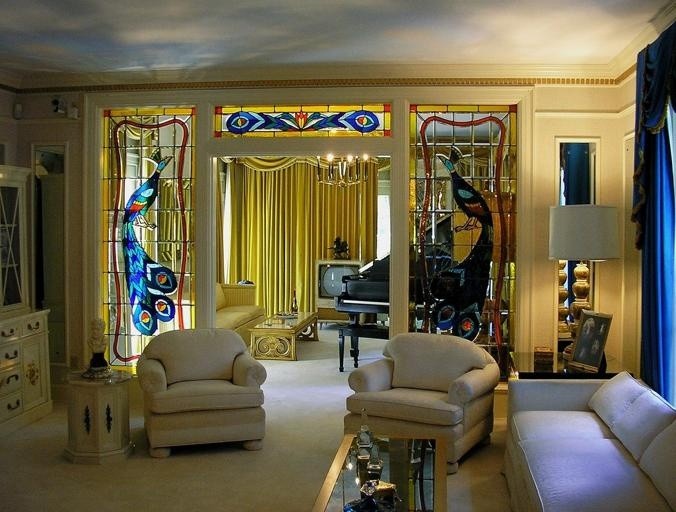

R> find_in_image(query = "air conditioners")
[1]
[14,92,87,120]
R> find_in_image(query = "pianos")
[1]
[334,214,451,372]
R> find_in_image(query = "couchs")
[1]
[507,376,676,512]
[214,283,265,349]
[136,328,266,459]
[343,333,500,476]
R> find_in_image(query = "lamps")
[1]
[317,155,369,188]
[549,203,623,362]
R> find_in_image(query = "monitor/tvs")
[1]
[315,259,364,308]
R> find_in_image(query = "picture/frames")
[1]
[569,309,612,373]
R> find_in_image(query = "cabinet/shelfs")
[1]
[0,166,53,437]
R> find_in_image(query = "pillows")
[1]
[609,389,676,462]
[638,418,676,511]
[590,372,648,429]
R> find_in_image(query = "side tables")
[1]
[62,371,135,466]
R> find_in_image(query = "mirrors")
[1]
[553,136,602,378]
[30,142,71,368]
